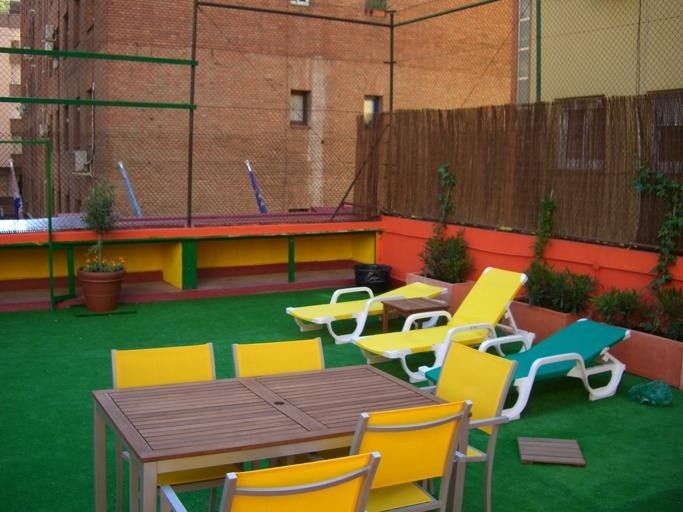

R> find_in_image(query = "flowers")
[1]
[75,179,128,271]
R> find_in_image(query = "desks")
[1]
[89,363,474,512]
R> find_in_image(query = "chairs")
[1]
[111,336,518,512]
[286,263,632,422]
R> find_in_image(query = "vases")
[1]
[77,266,127,312]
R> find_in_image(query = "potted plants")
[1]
[405,151,683,392]
